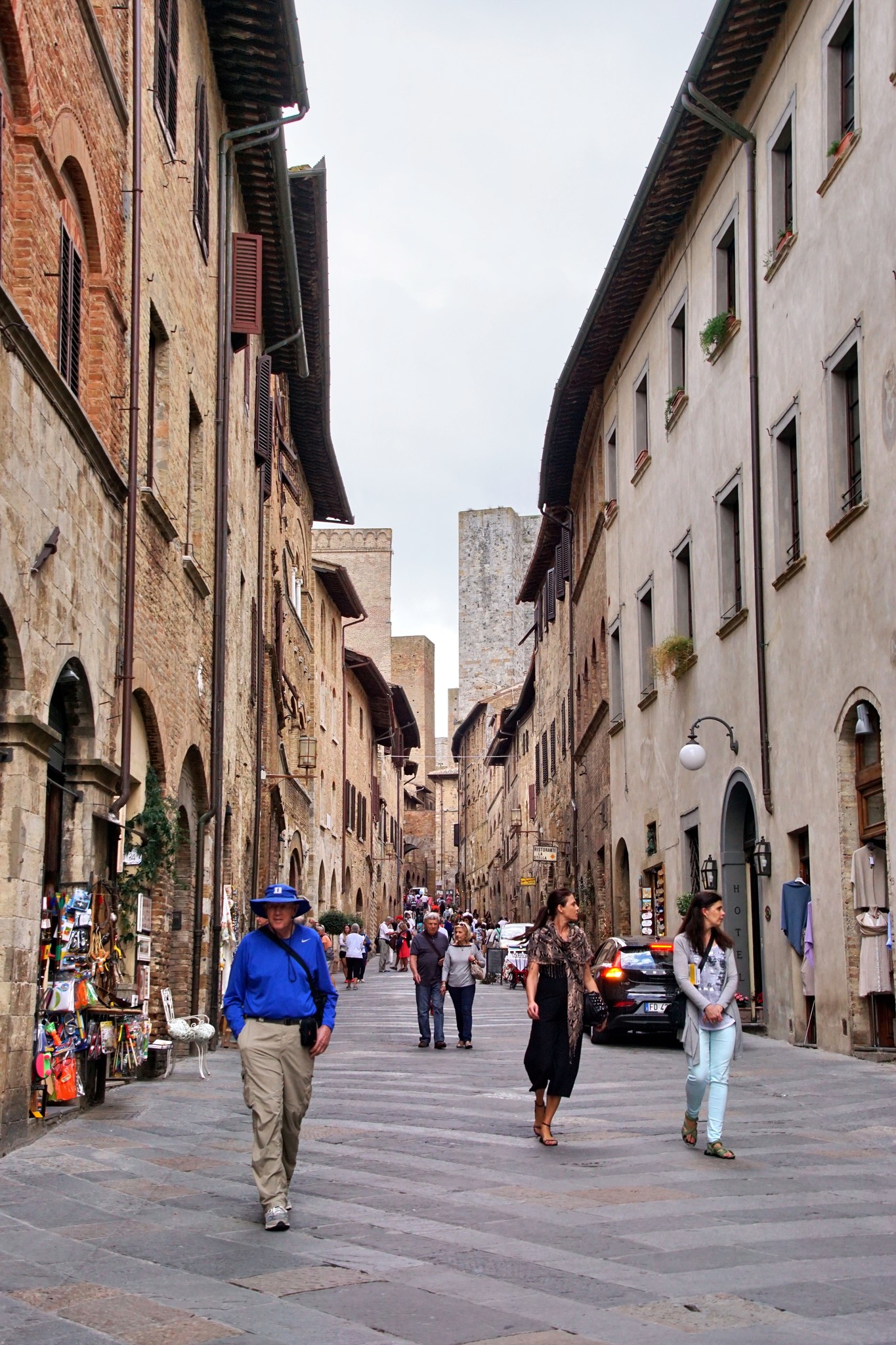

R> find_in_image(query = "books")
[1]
[688,964,701,985]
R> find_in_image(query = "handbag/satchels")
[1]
[583,990,608,1027]
[482,943,486,953]
[366,945,371,952]
[397,936,403,947]
[664,991,688,1030]
[313,990,327,1027]
[374,936,379,945]
[470,943,484,981]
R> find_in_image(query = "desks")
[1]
[88,1008,142,1104]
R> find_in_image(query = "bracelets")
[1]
[477,960,479,964]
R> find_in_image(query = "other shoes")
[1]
[336,990,338,993]
[465,1043,472,1049]
[456,1041,465,1047]
[379,962,408,973]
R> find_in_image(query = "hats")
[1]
[249,884,312,920]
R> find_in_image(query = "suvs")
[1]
[495,923,534,953]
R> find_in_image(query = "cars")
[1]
[583,936,681,1044]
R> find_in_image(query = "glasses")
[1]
[456,923,466,928]
[318,925,324,929]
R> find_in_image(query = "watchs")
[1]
[441,984,446,987]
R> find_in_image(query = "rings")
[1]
[713,1020,715,1022]
[472,957,473,959]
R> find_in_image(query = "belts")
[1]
[379,938,386,940]
[247,1015,300,1026]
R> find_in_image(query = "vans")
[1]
[409,887,427,895]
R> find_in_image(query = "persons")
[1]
[510,888,608,1146]
[379,887,510,972]
[440,922,485,1048]
[223,884,339,1231]
[295,916,371,994]
[257,915,269,927]
[430,1001,434,1015]
[673,891,743,1159]
[409,912,449,1047]
[855,905,894,997]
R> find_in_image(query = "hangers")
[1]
[782,870,811,890]
[853,838,886,859]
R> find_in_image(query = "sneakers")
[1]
[419,1041,429,1047]
[435,1041,446,1049]
[265,1206,290,1230]
[344,977,364,990]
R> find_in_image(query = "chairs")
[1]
[160,988,215,1079]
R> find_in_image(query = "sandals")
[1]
[533,1100,546,1136]
[705,1140,736,1159]
[681,1111,699,1146]
[540,1122,558,1146]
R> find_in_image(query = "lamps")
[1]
[493,858,511,881]
[448,855,461,868]
[424,851,429,858]
[510,808,543,842]
[415,856,425,864]
[373,842,396,872]
[753,836,771,878]
[700,855,718,891]
[679,716,738,771]
[261,737,318,810]
[404,854,413,870]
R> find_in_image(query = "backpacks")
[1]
[440,900,445,906]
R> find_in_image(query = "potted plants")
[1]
[319,909,347,974]
[339,914,364,972]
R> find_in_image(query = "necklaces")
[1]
[458,941,467,950]
[868,911,880,919]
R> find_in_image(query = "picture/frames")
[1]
[137,934,151,962]
